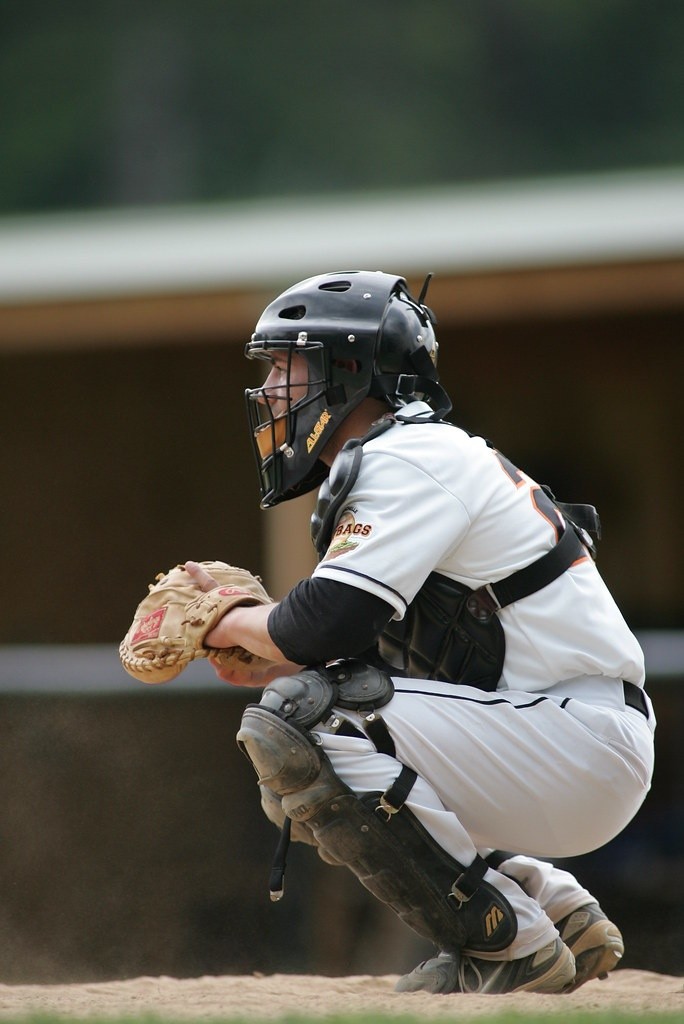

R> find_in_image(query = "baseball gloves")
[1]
[117,558,278,686]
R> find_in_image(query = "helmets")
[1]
[245,271,441,509]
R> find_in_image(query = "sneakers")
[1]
[395,926,575,994]
[555,903,623,995]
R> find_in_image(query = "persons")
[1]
[143,269,659,994]
